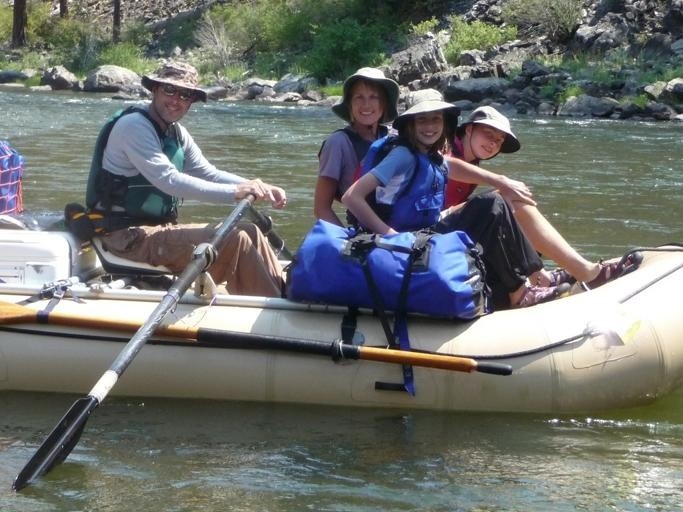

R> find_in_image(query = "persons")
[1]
[342,89,576,307]
[86,61,286,298]
[311,67,642,293]
[443,105,542,314]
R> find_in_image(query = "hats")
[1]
[459,105,520,153]
[393,88,460,131]
[141,62,207,104]
[331,67,399,123]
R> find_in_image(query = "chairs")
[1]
[88,232,173,280]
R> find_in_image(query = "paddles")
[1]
[13,192,256,491]
[0,298,513,376]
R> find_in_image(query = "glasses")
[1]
[156,81,193,100]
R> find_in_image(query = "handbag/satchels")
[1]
[288,219,490,319]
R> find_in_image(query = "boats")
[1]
[0,209,683,414]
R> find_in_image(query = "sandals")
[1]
[514,282,569,308]
[549,267,576,287]
[584,252,643,291]
